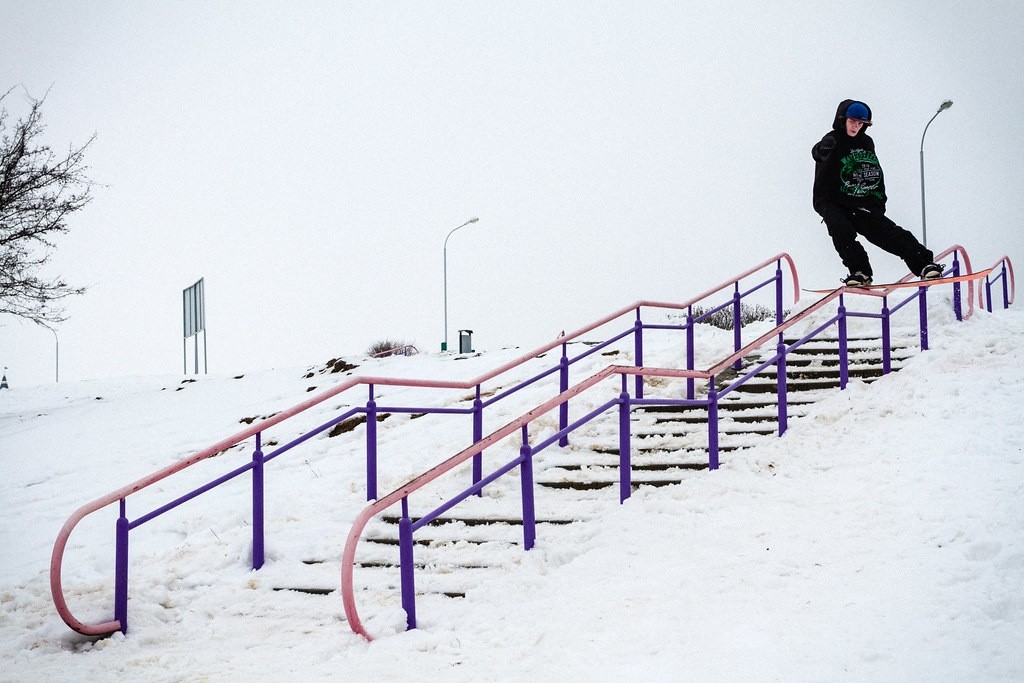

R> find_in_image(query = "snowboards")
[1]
[798,267,993,294]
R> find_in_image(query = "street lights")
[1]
[441,218,482,351]
[920,100,954,249]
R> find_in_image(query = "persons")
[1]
[810,99,943,286]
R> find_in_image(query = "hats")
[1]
[845,102,870,123]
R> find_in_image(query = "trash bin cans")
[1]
[458,330,473,353]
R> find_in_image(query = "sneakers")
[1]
[839,270,873,289]
[920,262,946,281]
